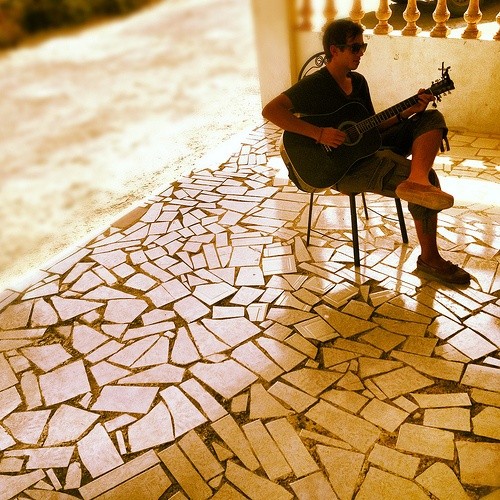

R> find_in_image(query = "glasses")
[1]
[333,44,368,53]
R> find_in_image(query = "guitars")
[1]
[279,73,455,191]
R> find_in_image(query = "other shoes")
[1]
[395,181,455,210]
[417,253,470,283]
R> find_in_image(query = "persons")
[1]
[262,18,471,285]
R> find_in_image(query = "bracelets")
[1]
[396,113,409,123]
[316,127,323,145]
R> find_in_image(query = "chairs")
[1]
[297,52,408,266]
